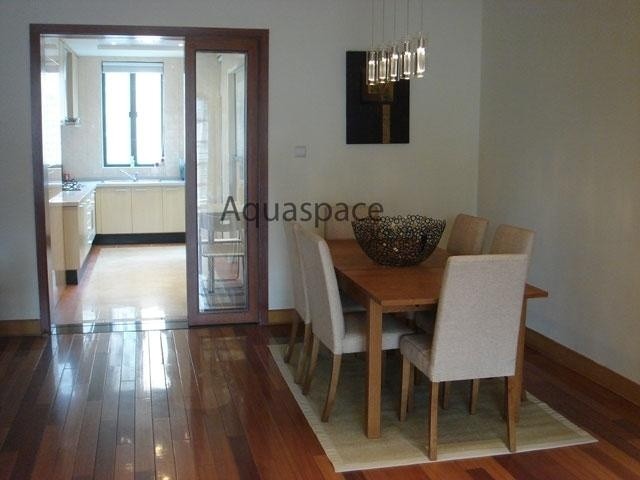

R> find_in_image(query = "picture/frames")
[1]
[346,51,411,144]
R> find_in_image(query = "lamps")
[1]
[388,3,400,83]
[416,3,427,79]
[403,3,413,82]
[378,4,388,86]
[368,3,376,86]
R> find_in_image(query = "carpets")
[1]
[266,343,598,474]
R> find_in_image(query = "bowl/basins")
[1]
[351,214,447,268]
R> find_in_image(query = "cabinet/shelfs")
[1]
[49,181,96,285]
[132,185,185,244]
[96,185,132,244]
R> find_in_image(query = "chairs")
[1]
[446,214,488,257]
[290,227,414,422]
[400,252,524,462]
[283,222,365,382]
[202,214,247,293]
[487,224,532,268]
